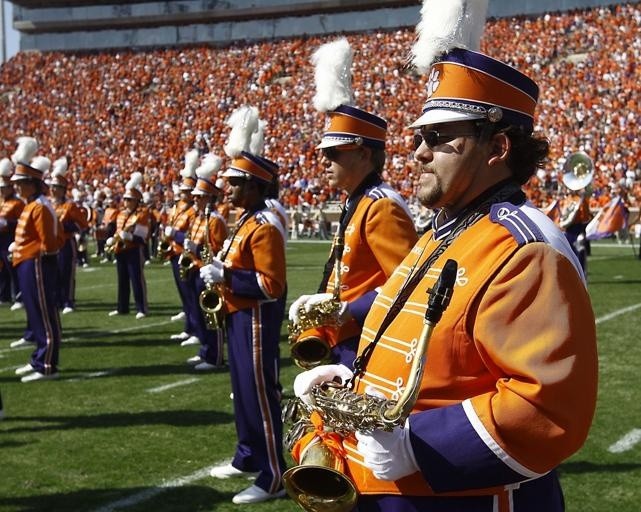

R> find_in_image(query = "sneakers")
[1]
[109,310,120,317]
[135,312,145,320]
[62,307,74,314]
[15,363,57,383]
[9,338,37,348]
[232,483,286,504]
[11,301,24,310]
[171,312,188,321]
[170,331,221,370]
[210,463,263,480]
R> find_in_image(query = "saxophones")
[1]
[103,215,139,253]
[178,208,200,283]
[280,256,459,512]
[157,205,178,264]
[287,209,346,369]
[200,203,223,334]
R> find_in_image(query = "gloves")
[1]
[355,417,421,482]
[119,231,133,241]
[183,238,198,255]
[294,364,354,407]
[199,256,224,285]
[288,293,349,323]
[165,227,176,237]
[106,237,116,246]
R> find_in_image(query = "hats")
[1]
[405,47,539,129]
[0,162,42,188]
[220,151,279,184]
[179,175,221,197]
[122,187,142,201]
[314,104,388,150]
[48,175,67,188]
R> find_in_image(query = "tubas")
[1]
[558,151,595,227]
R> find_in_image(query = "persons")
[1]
[296,111,597,512]
[208,162,286,503]
[1,0,641,381]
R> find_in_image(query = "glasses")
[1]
[321,149,351,159]
[413,130,478,149]
